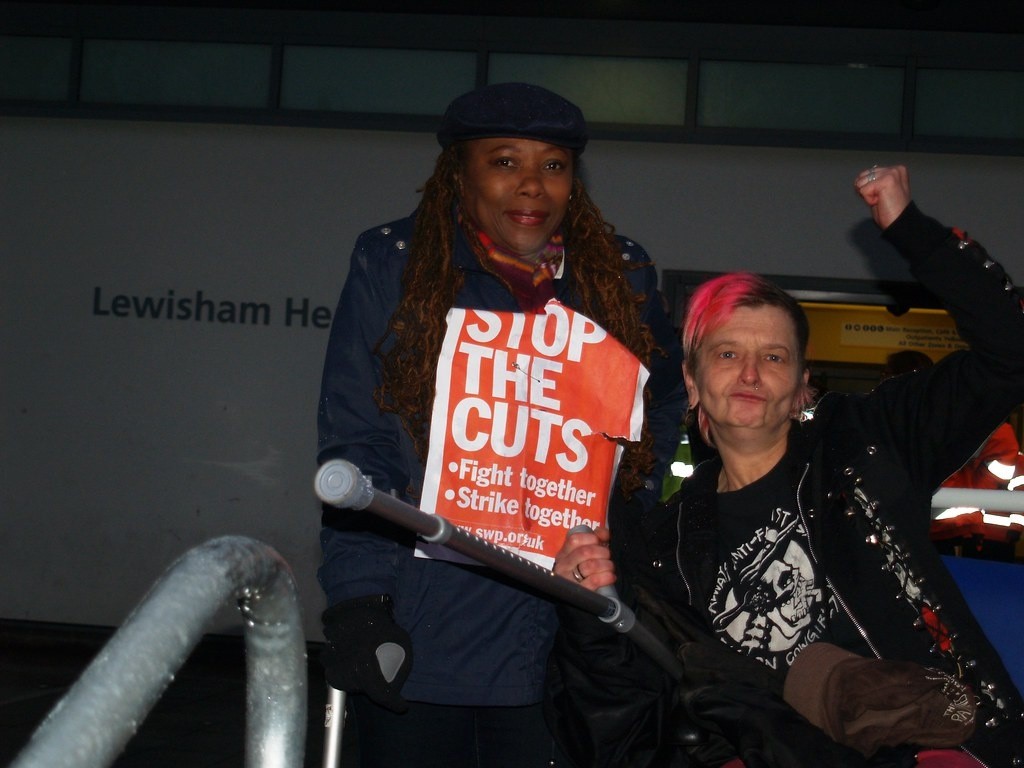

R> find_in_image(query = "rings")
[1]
[869,167,875,173]
[573,565,585,582]
[868,174,875,181]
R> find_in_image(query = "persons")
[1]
[548,165,1024,768]
[317,82,686,768]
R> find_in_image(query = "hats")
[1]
[436,82,590,157]
[781,642,977,757]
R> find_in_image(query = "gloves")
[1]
[320,595,413,717]
